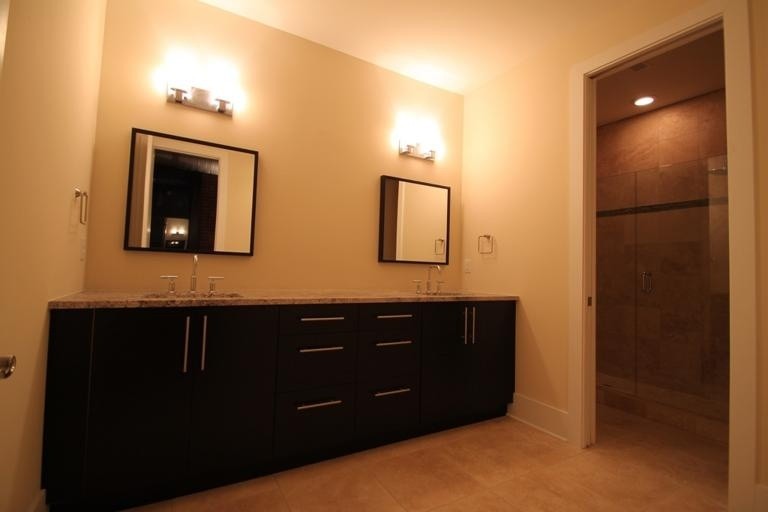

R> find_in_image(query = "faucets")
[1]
[190,254,199,293]
[427,264,441,293]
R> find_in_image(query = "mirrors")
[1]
[121,124,260,256]
[376,173,452,268]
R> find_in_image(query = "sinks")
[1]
[142,292,242,300]
[424,292,467,296]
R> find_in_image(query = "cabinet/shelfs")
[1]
[594,154,730,447]
[41,299,521,511]
